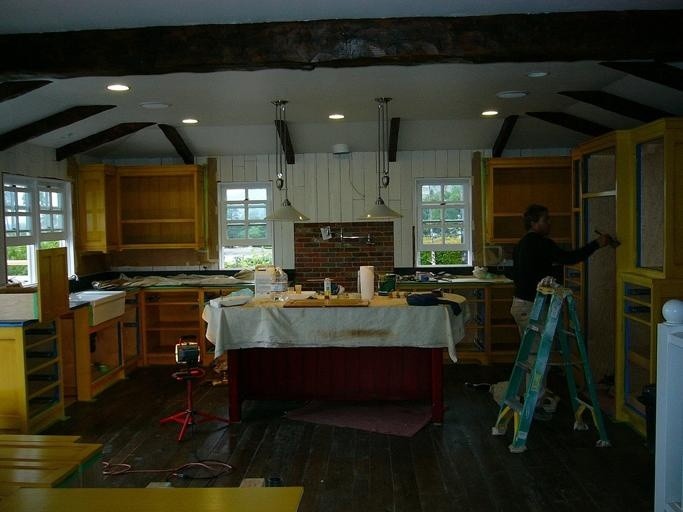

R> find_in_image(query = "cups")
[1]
[288,285,301,294]
[420,274,428,281]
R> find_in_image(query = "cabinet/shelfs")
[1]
[76,162,207,254]
[487,157,578,362]
[398,284,487,366]
[58,290,143,402]
[144,290,254,366]
[0,316,71,435]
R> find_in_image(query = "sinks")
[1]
[69,289,127,326]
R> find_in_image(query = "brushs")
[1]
[594,230,621,248]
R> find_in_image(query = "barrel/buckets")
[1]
[255,264,275,297]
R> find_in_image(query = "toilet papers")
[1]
[359,265,375,301]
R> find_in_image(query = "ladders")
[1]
[492,286,611,453]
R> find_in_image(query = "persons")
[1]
[509,204,614,423]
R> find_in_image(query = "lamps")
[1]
[356,97,402,221]
[264,100,311,223]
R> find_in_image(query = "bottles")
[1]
[323,277,331,299]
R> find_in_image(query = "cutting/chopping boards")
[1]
[283,299,369,307]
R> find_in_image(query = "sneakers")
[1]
[533,407,553,422]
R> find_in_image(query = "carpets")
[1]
[285,402,448,438]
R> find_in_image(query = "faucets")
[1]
[69,274,80,281]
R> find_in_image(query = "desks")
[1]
[0,433,108,487]
[205,292,449,426]
[2,487,304,511]
